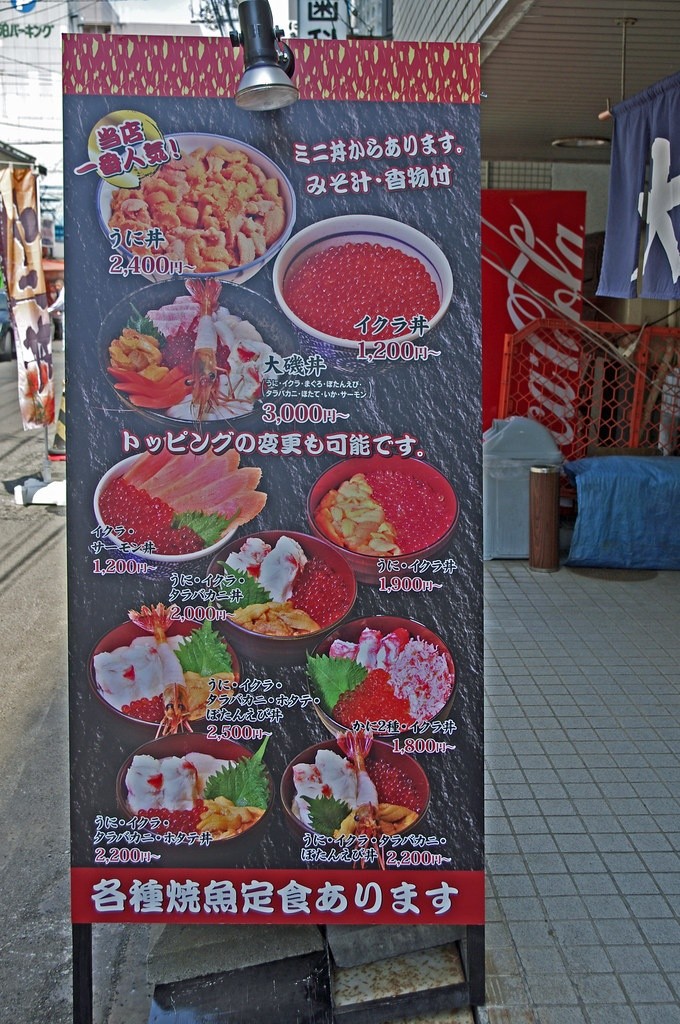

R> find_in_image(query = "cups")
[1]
[14,487,27,504]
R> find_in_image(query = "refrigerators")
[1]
[481,189,585,489]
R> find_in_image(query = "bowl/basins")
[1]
[92,453,237,584]
[96,132,297,286]
[272,214,453,377]
[306,454,459,586]
[207,531,356,666]
[87,616,244,726]
[280,737,430,847]
[115,732,274,845]
[97,279,297,433]
[306,615,458,748]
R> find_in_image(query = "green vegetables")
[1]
[172,615,233,677]
[217,560,274,615]
[304,649,369,717]
[125,302,167,349]
[172,508,241,549]
[299,795,352,838]
[202,734,270,809]
